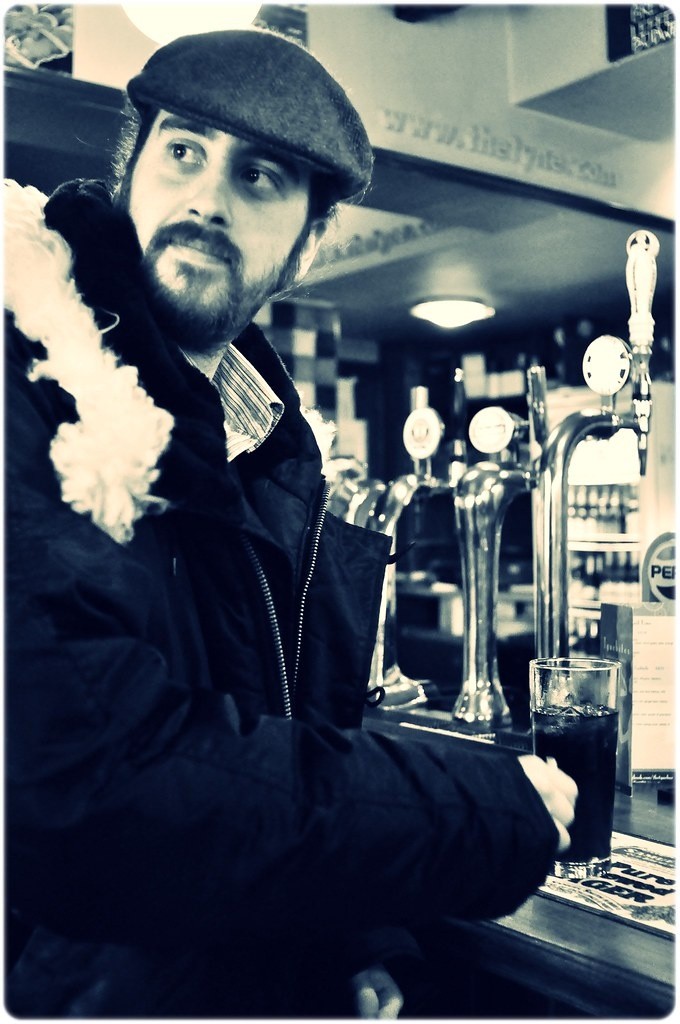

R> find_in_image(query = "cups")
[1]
[528,659,622,877]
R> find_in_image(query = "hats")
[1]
[126,30,373,201]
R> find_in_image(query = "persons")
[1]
[5,29,576,1020]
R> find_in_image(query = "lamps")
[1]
[408,293,496,330]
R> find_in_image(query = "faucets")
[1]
[367,387,467,709]
[454,366,549,722]
[534,346,653,708]
[323,455,368,524]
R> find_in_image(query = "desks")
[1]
[381,707,674,1020]
